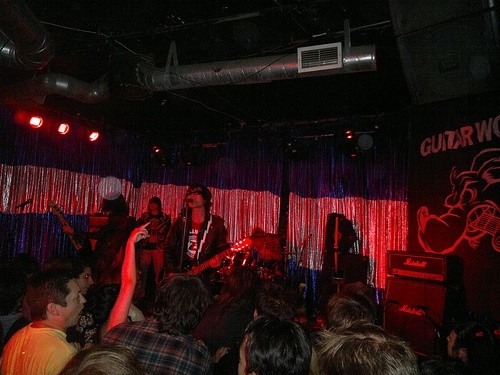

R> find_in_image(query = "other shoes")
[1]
[133,299,141,305]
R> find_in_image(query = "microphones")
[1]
[15,199,32,208]
[186,197,195,202]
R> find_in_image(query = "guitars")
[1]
[184,236,252,276]
[48,199,94,269]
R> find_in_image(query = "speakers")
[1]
[384,275,448,360]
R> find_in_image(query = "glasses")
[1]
[187,191,204,195]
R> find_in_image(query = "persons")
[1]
[136,196,171,291]
[192,267,263,358]
[325,281,381,330]
[97,273,213,375]
[427,322,500,375]
[65,222,152,350]
[63,192,135,285]
[238,314,312,375]
[164,183,229,312]
[0,272,87,375]
[312,324,419,375]
